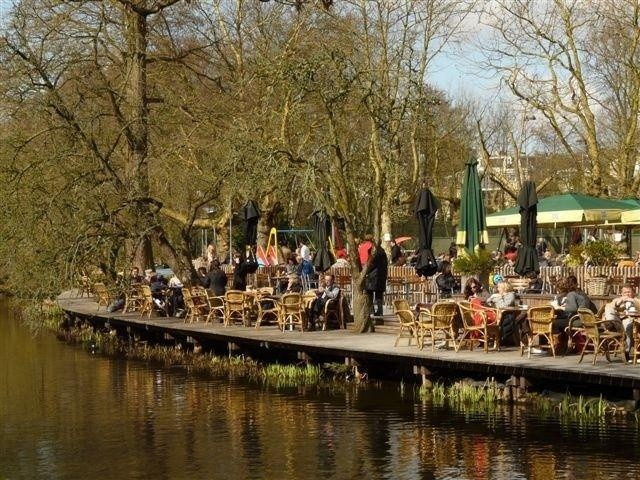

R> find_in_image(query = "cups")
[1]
[514,299,519,308]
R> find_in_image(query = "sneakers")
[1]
[530,346,549,354]
[627,357,640,364]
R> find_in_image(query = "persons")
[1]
[197,260,228,296]
[437,260,460,293]
[306,272,341,332]
[390,239,407,266]
[551,275,598,355]
[261,272,303,327]
[232,251,248,291]
[281,236,312,273]
[605,283,638,356]
[358,234,388,313]
[464,278,531,348]
[128,267,185,318]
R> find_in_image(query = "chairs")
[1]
[73,267,640,366]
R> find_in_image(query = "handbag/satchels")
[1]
[588,299,598,315]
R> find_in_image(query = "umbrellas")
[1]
[413,181,439,277]
[455,154,489,260]
[514,181,540,276]
[240,200,261,274]
[310,208,336,271]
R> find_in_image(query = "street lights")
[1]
[520,114,541,195]
